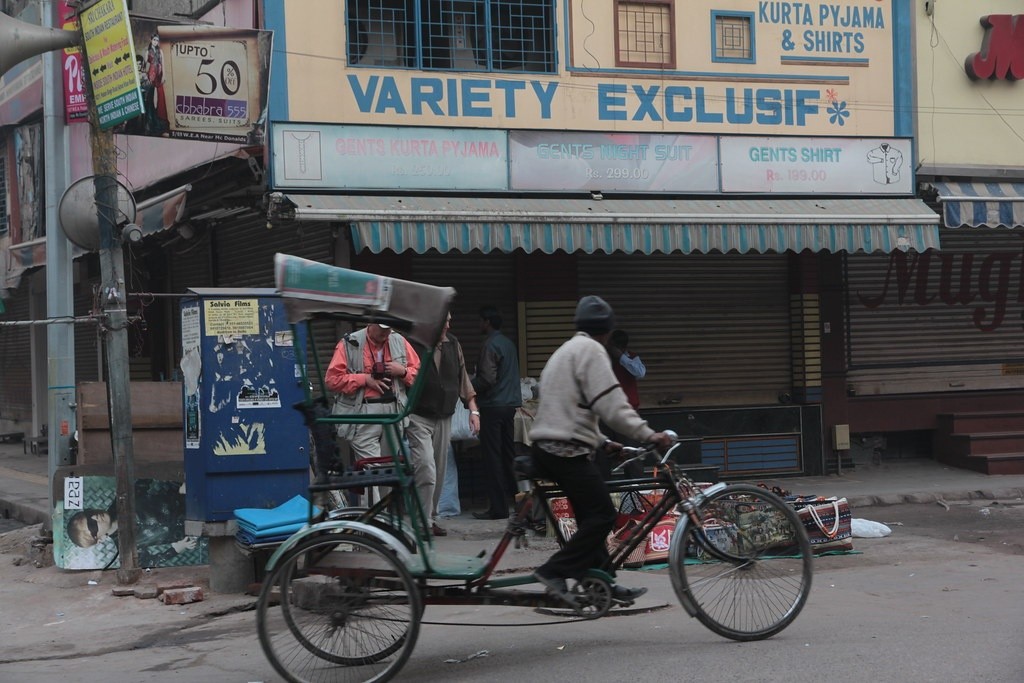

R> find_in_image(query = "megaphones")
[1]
[0,10,80,77]
[58,174,143,245]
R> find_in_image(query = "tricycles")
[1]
[181,252,815,683]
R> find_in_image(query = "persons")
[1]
[406,310,482,542]
[471,308,522,520]
[325,323,421,509]
[69,480,199,564]
[606,330,647,477]
[528,294,671,609]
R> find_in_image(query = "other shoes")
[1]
[594,585,648,612]
[433,523,447,536]
[479,510,509,519]
[418,527,434,540]
[475,498,491,518]
[533,562,582,607]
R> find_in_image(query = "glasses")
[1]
[85,508,99,543]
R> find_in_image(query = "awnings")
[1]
[923,183,1023,231]
[286,194,943,255]
[9,188,188,272]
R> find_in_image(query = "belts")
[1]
[362,398,397,403]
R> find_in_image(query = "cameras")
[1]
[372,362,389,380]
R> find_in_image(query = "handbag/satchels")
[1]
[559,483,854,569]
[450,395,480,439]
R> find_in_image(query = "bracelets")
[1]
[403,368,408,377]
[470,411,481,416]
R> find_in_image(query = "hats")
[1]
[573,295,616,336]
